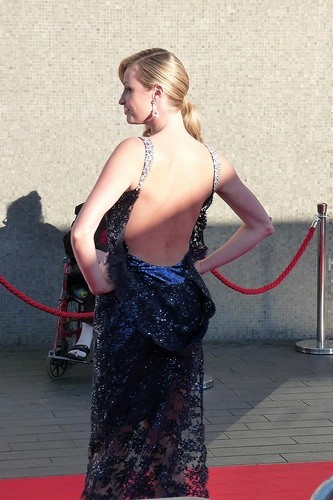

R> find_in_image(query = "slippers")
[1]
[66,345,90,361]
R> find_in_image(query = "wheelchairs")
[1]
[45,257,96,380]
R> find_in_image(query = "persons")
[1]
[68,46,276,500]
[63,203,111,359]
